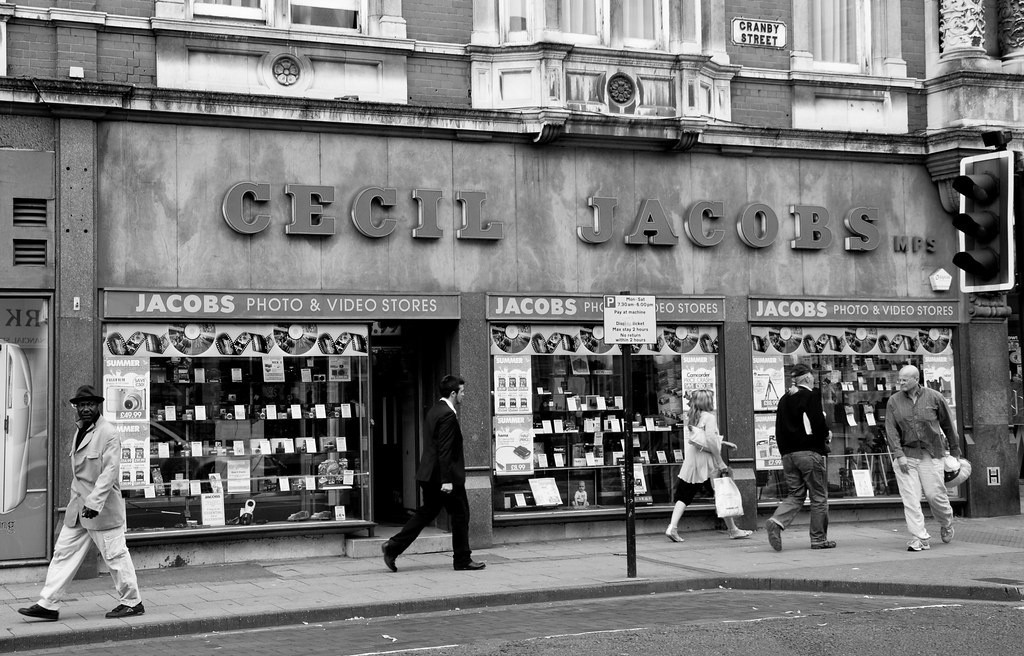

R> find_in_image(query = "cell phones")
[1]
[122,448,145,482]
[498,376,528,409]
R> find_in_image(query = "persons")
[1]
[665,389,752,543]
[884,366,962,552]
[571,481,589,510]
[382,374,485,572]
[18,385,145,620]
[765,364,836,552]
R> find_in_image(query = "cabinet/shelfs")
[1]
[755,355,969,505]
[110,353,378,537]
[493,356,718,519]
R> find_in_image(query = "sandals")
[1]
[729,529,752,539]
[666,524,684,542]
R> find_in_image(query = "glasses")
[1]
[896,378,915,383]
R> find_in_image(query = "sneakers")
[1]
[940,509,954,543]
[17,604,59,621]
[907,540,930,551]
[106,602,144,617]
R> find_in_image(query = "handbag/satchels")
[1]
[713,472,743,518]
[687,424,723,453]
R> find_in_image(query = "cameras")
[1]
[153,374,343,496]
[332,368,348,377]
[105,385,146,413]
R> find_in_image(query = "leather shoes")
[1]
[381,540,399,573]
[767,519,782,551]
[812,540,836,549]
[454,561,485,569]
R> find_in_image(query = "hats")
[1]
[70,385,105,405]
[791,364,814,377]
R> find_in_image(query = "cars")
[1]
[21,414,279,490]
[249,459,369,520]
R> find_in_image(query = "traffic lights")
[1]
[951,150,1016,293]
